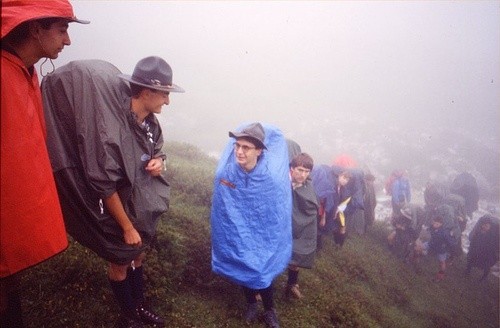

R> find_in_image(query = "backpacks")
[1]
[432,204,461,247]
[384,168,407,194]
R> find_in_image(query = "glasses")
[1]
[233,143,257,151]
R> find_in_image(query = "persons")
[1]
[253,138,321,304]
[463,216,500,286]
[309,154,377,260]
[87,56,185,328]
[209,122,294,328]
[384,168,480,283]
[0,0,79,328]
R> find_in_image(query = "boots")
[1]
[317,234,322,249]
[333,230,347,252]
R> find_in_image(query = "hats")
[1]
[118,57,184,93]
[228,122,267,149]
[400,207,412,220]
[68,16,91,25]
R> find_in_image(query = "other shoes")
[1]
[286,287,303,300]
[119,308,149,328]
[436,270,446,279]
[245,303,260,328]
[263,308,281,328]
[255,292,263,302]
[135,299,165,328]
